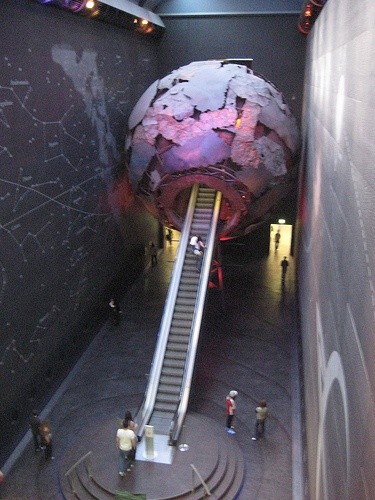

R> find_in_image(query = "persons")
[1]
[108,296,122,330]
[29,410,46,451]
[275,230,280,253]
[208,255,223,318]
[280,256,289,280]
[117,410,139,467]
[225,391,238,434]
[191,234,207,273]
[115,418,136,477]
[168,229,172,245]
[148,241,158,267]
[39,419,56,460]
[252,399,272,440]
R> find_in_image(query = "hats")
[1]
[229,390,238,397]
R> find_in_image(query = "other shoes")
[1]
[227,428,236,434]
[252,437,257,441]
[119,464,134,476]
